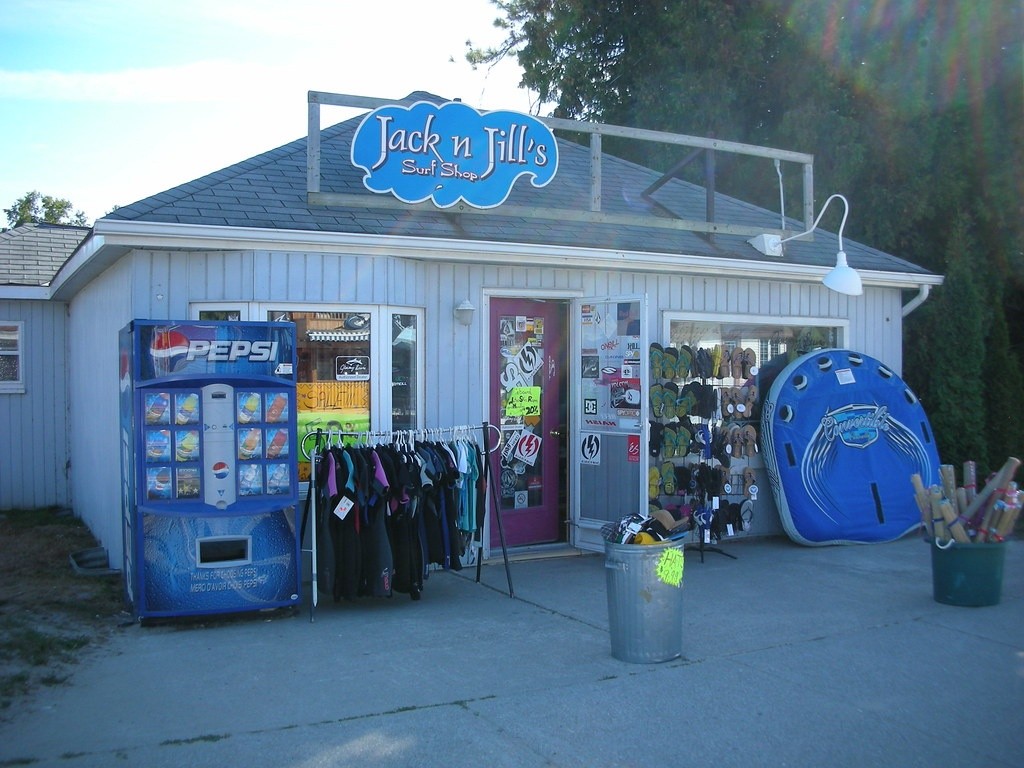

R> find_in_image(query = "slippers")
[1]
[648,338,760,538]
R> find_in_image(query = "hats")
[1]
[600,508,691,545]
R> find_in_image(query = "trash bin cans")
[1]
[603,535,689,664]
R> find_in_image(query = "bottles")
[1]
[177,431,197,461]
[239,393,260,423]
[267,428,287,457]
[239,429,260,459]
[148,468,170,499]
[145,393,168,424]
[177,394,198,424]
[239,464,257,495]
[268,464,286,494]
[267,394,287,422]
[147,430,169,462]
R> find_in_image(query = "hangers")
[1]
[323,425,477,450]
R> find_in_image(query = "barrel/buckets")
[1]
[924,536,1009,606]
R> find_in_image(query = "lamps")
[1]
[454,298,475,327]
[751,194,864,296]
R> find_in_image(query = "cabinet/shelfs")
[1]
[650,362,752,562]
